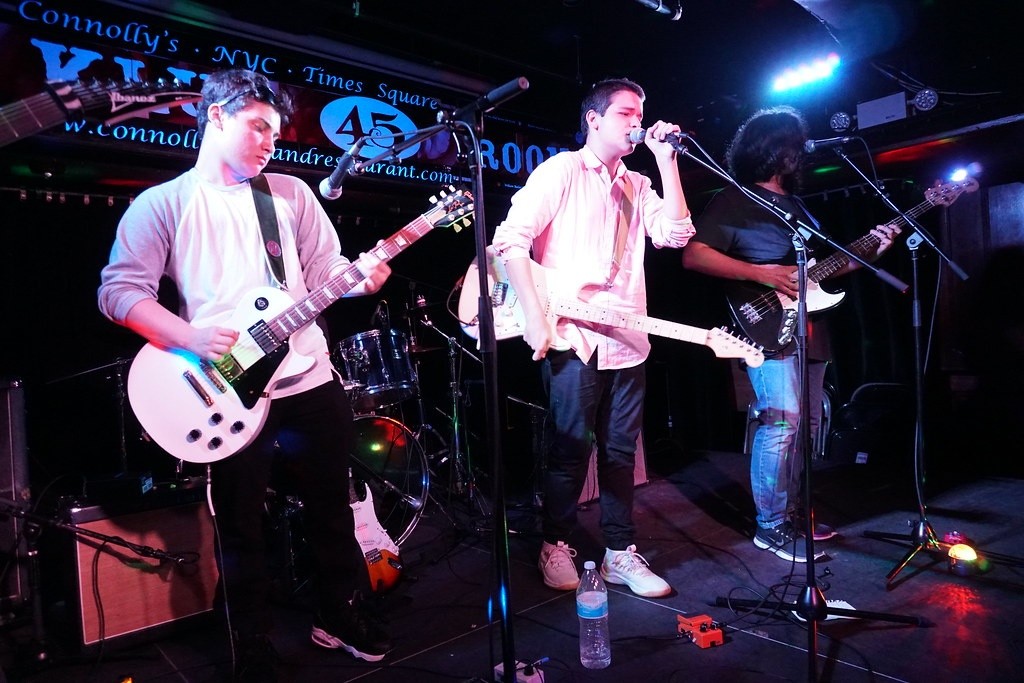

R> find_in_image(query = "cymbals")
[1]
[408,344,447,354]
[42,357,130,384]
[395,300,453,323]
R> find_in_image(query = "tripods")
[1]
[405,313,526,566]
[667,137,1024,683]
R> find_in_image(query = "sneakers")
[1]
[790,515,838,540]
[600,544,671,597]
[310,604,396,661]
[752,520,826,562]
[538,538,581,590]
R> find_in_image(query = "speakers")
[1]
[0,376,34,607]
[45,476,217,654]
[579,431,647,501]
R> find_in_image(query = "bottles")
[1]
[576,560,610,667]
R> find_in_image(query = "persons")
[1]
[492,77,696,598]
[97,68,392,683]
[682,111,902,562]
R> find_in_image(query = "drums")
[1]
[348,414,432,548]
[332,327,419,412]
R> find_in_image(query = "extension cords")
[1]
[495,659,545,683]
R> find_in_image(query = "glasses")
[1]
[217,82,293,109]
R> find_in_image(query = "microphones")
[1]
[319,138,366,200]
[629,127,689,145]
[173,556,186,568]
[371,301,385,326]
[803,136,856,154]
[402,495,424,511]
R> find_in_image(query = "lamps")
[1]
[830,81,944,140]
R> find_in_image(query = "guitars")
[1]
[126,183,477,465]
[346,463,402,596]
[458,245,767,369]
[725,175,982,359]
